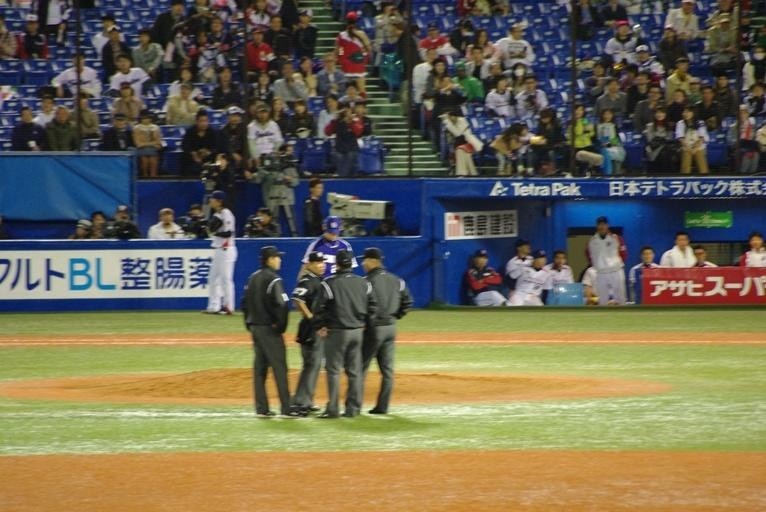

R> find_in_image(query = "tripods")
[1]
[201,194,213,220]
[267,185,299,237]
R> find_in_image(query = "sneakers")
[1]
[257,403,386,419]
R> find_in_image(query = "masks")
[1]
[514,69,525,77]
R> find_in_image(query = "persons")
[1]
[286,250,327,415]
[464,238,614,306]
[295,216,360,286]
[370,218,403,237]
[731,233,766,267]
[629,233,719,305]
[585,216,629,306]
[336,218,367,236]
[308,249,379,419]
[69,154,325,315]
[372,1,766,177]
[2,0,387,177]
[238,242,309,418]
[354,246,414,414]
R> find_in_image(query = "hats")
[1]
[100,12,121,32]
[298,8,313,17]
[308,251,326,261]
[76,205,174,230]
[427,21,440,30]
[351,95,374,106]
[112,81,155,121]
[346,10,362,20]
[323,215,341,234]
[261,244,287,255]
[700,77,712,91]
[205,190,226,201]
[616,0,731,63]
[474,238,547,260]
[181,81,192,89]
[228,104,271,115]
[335,250,353,262]
[25,15,39,22]
[356,247,384,259]
